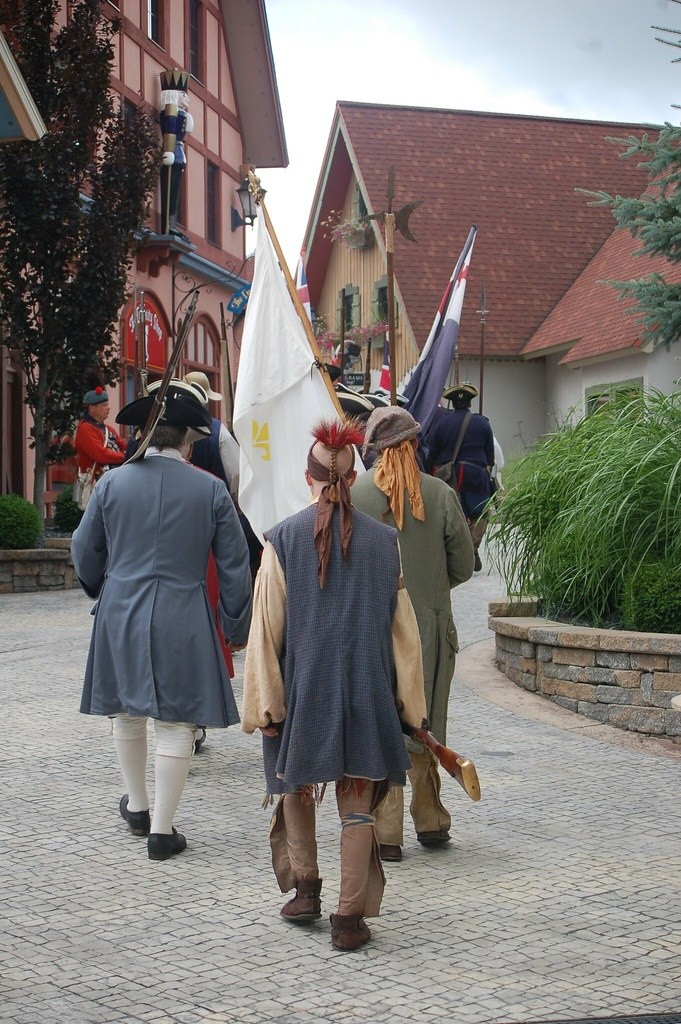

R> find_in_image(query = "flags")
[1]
[232,205,367,550]
[296,248,312,325]
[394,224,477,449]
[378,326,391,390]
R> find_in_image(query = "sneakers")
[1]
[147,826,187,860]
[330,913,371,951]
[120,794,151,835]
[280,878,323,923]
[381,845,402,860]
[417,831,452,846]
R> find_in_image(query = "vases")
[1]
[343,235,371,249]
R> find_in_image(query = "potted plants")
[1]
[0,485,87,593]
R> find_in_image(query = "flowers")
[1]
[320,209,373,249]
[314,314,389,350]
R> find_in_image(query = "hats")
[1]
[114,372,222,445]
[325,363,342,382]
[83,387,109,404]
[362,406,423,460]
[442,384,478,398]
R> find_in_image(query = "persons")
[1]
[330,383,506,571]
[74,372,263,749]
[343,406,475,860]
[240,422,428,953]
[68,386,254,861]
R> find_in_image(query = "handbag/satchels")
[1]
[434,462,457,488]
[72,473,97,511]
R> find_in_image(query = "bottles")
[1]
[73,476,81,502]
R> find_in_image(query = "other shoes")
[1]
[474,550,482,571]
[195,729,206,752]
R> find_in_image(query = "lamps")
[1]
[231,176,267,232]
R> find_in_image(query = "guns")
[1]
[364,336,372,393]
[400,716,483,803]
[132,287,141,441]
[133,288,201,460]
[219,300,234,433]
